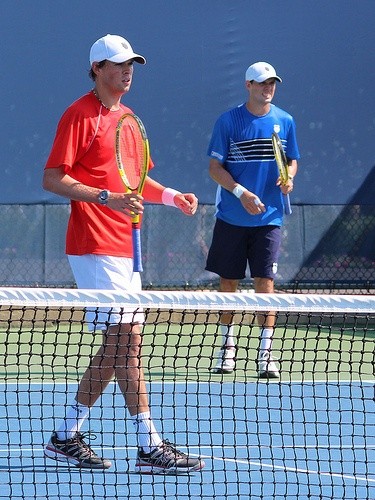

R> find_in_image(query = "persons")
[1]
[43,33,205,475]
[337,203,375,257]
[204,62,301,378]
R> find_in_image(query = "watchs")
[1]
[98,189,110,205]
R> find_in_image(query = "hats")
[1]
[245,61,282,84]
[89,33,147,65]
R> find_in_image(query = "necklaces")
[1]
[91,88,119,111]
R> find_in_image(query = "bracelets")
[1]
[287,174,294,180]
[161,187,182,208]
[232,184,247,198]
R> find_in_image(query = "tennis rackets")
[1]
[272,132,293,216]
[115,111,151,273]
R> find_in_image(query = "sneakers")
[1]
[257,352,280,378]
[44,430,111,469]
[135,438,206,475]
[213,350,235,373]
